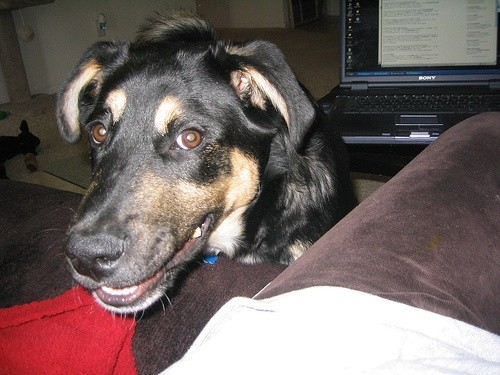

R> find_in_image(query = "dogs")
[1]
[57,9,360,331]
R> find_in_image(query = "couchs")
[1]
[0,180,290,375]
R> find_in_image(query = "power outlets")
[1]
[96,20,106,37]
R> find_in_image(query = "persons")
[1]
[158,109,500,375]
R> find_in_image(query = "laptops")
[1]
[328,0,500,144]
